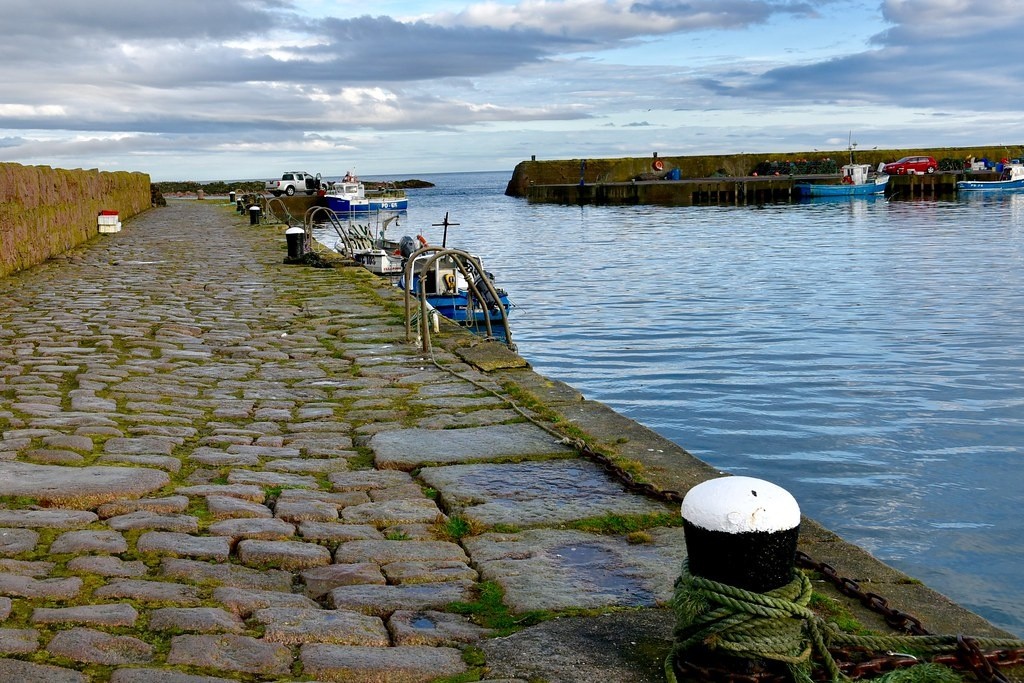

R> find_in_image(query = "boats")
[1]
[792,165,890,196]
[334,199,428,275]
[397,212,510,327]
[791,191,884,204]
[956,163,1024,191]
[315,166,408,215]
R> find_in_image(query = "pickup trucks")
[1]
[265,171,329,198]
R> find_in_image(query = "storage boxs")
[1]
[97,211,121,233]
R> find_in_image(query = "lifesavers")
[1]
[653,160,663,170]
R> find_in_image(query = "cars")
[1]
[882,156,938,175]
